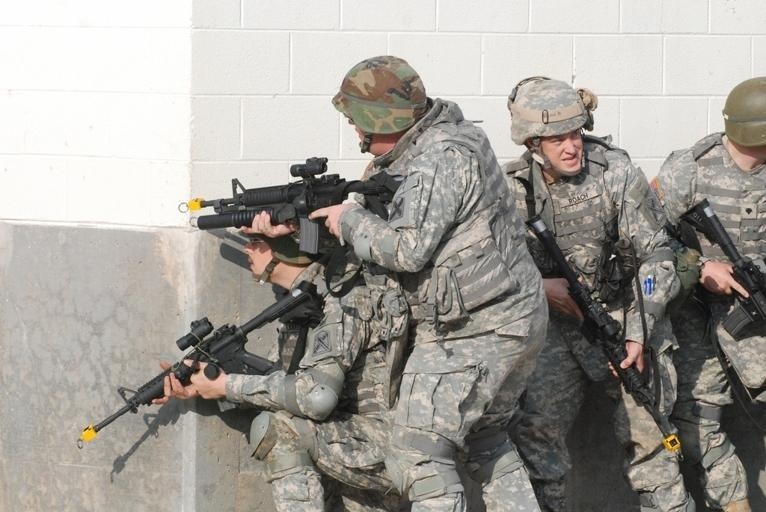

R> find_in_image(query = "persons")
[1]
[152,57,764,512]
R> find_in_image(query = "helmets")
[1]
[723,77,766,146]
[508,77,588,145]
[238,230,317,264]
[332,56,428,134]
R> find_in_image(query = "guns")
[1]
[178,157,402,254]
[680,198,765,337]
[77,281,323,440]
[523,214,684,462]
